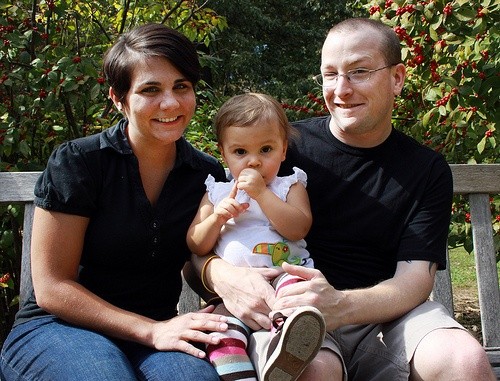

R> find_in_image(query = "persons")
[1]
[192,19,498,381]
[1,25,229,381]
[186,92,327,381]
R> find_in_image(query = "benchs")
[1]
[0,164,500,381]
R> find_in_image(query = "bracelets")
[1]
[201,255,221,294]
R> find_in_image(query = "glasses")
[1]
[312,63,400,87]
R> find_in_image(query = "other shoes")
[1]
[261,306,326,381]
